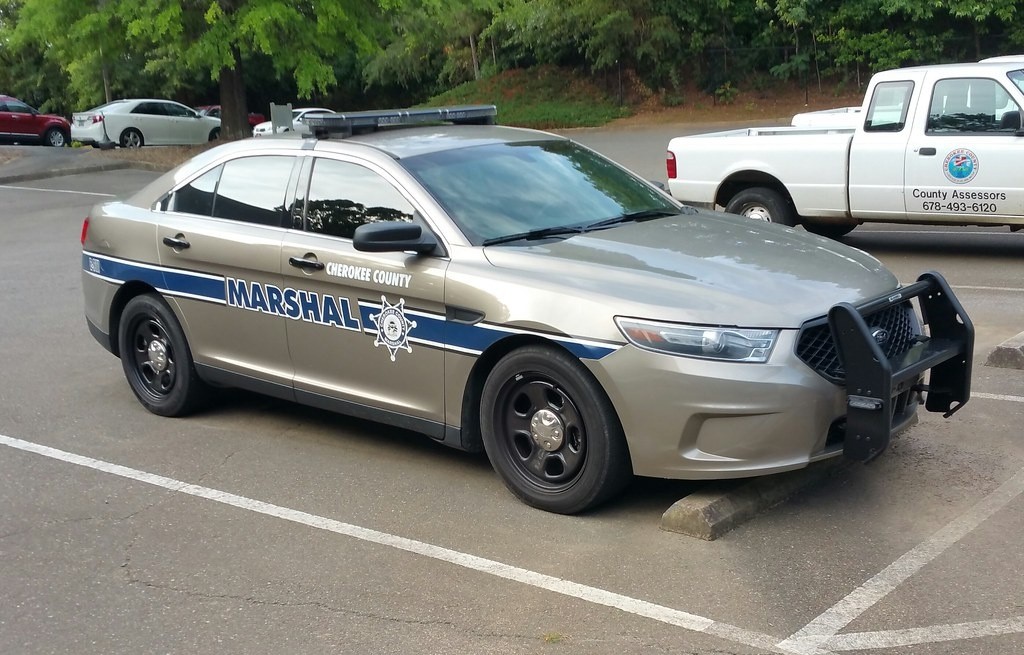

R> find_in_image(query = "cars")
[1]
[69,97,222,150]
[78,102,976,517]
[194,104,267,132]
[252,107,337,138]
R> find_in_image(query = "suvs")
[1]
[0,92,71,148]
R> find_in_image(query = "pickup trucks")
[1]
[791,55,1024,127]
[664,62,1023,241]
